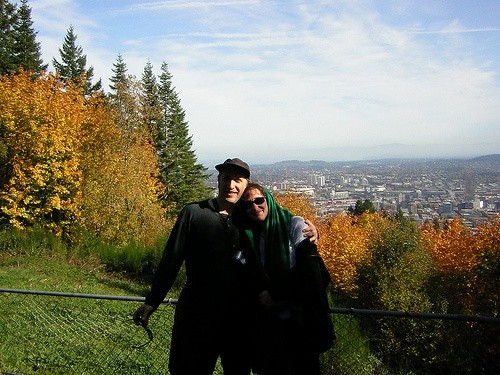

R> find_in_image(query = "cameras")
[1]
[234,250,247,265]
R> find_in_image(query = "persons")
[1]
[237,182,338,375]
[133,158,319,375]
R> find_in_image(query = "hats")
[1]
[215,158,251,179]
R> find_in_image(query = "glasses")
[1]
[119,317,153,348]
[240,197,265,210]
